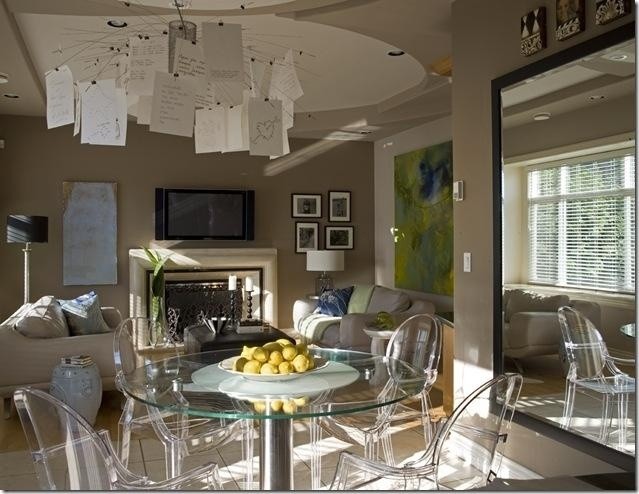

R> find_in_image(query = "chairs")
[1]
[309,311,446,490]
[556,303,635,452]
[0,298,137,422]
[112,315,258,490]
[12,384,224,493]
[326,373,526,492]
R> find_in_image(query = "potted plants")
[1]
[135,242,179,347]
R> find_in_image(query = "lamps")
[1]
[305,249,347,301]
[5,213,49,304]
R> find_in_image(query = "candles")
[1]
[226,274,237,289]
[245,274,254,292]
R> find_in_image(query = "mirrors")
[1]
[489,19,637,472]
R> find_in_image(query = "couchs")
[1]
[500,284,602,379]
[293,283,437,373]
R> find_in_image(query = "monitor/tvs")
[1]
[154,187,255,241]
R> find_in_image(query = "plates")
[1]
[217,353,329,380]
[218,376,329,399]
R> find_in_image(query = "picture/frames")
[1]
[327,188,353,222]
[323,224,355,250]
[289,191,324,218]
[294,219,320,254]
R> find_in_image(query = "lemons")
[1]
[249,396,307,415]
[232,338,315,374]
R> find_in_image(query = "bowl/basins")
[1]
[202,316,231,333]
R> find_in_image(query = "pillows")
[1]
[314,287,355,315]
[60,289,96,308]
[503,288,571,324]
[60,293,111,336]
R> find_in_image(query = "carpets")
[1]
[500,356,582,399]
[0,380,442,458]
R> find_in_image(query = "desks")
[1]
[361,325,412,388]
[119,346,428,493]
[617,320,635,339]
